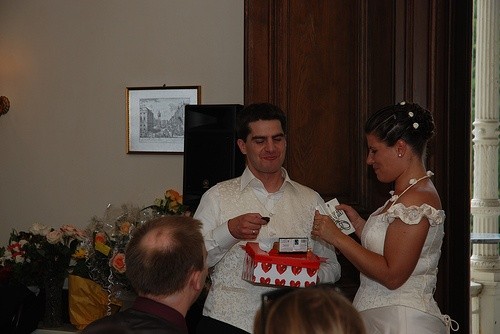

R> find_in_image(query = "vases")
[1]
[43,277,65,328]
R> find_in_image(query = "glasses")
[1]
[260,282,344,334]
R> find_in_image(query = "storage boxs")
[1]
[241,241,320,288]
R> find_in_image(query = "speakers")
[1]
[182,104,246,209]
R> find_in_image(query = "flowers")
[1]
[0,190,194,294]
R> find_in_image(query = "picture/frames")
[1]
[125,86,201,155]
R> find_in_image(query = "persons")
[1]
[253,288,367,334]
[310,102,461,334]
[191,103,342,334]
[80,215,210,334]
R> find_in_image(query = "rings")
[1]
[252,230,256,234]
[314,225,318,231]
